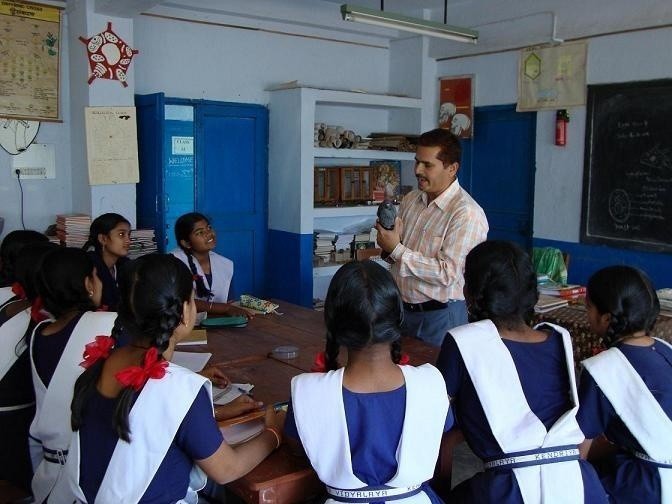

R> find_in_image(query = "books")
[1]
[170,326,290,449]
[312,225,384,262]
[41,211,161,261]
[652,287,672,321]
[373,187,388,204]
[531,272,589,313]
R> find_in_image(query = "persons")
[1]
[574,262,672,504]
[27,241,122,504]
[77,212,137,311]
[42,250,294,503]
[0,228,56,452]
[282,258,454,504]
[171,210,259,321]
[374,126,492,351]
[431,235,613,504]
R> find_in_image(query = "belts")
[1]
[403,299,448,312]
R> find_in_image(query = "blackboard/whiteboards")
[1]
[579,77,671,255]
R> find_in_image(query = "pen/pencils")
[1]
[231,384,254,399]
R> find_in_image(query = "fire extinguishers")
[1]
[555,109,569,147]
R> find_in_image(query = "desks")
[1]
[529,299,671,370]
[170,297,466,503]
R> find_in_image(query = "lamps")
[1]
[340,0,479,44]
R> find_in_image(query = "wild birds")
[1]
[376,197,401,260]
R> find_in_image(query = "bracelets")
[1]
[264,424,282,450]
[207,301,219,315]
[211,406,221,421]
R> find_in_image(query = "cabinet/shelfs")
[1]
[134,92,270,307]
[262,79,424,310]
